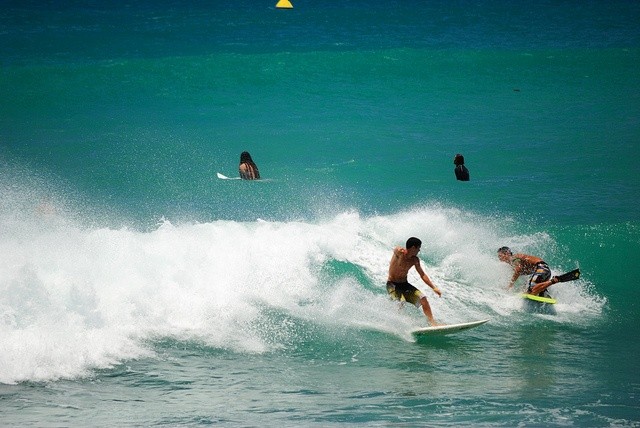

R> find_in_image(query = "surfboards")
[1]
[216,172,231,180]
[524,293,555,304]
[410,317,490,335]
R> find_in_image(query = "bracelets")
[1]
[434,288,438,292]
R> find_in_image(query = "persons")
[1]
[497,247,561,303]
[386,237,446,328]
[454,154,469,181]
[239,152,260,181]
